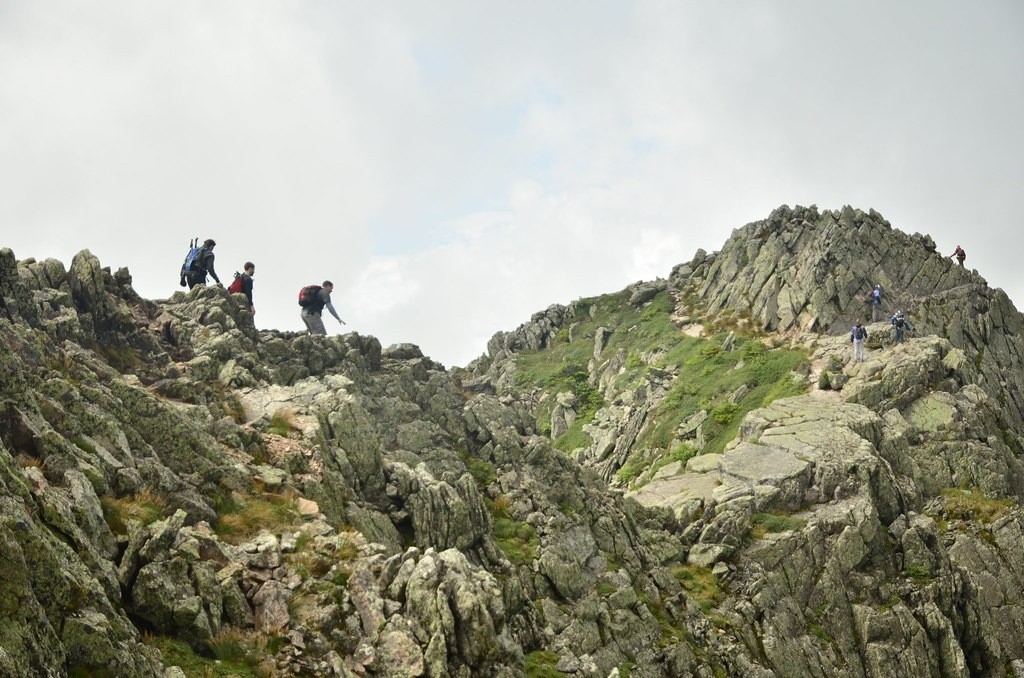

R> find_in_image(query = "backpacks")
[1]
[228,271,245,294]
[182,247,208,275]
[298,285,323,307]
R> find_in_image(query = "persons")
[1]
[871,284,882,322]
[241,262,256,315]
[850,319,868,361]
[890,311,910,345]
[179,239,223,291]
[951,245,966,266]
[300,281,346,335]
